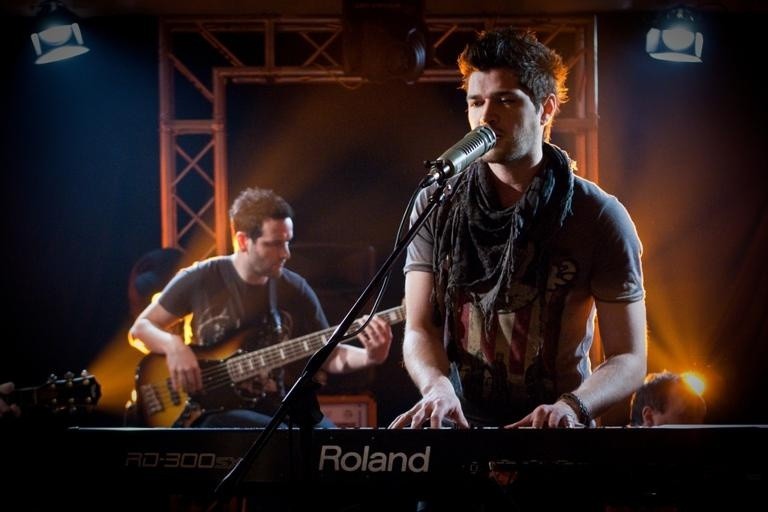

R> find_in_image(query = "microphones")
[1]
[420,126,497,188]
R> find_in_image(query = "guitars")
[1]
[1,368,101,423]
[134,305,403,427]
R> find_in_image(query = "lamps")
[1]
[642,2,706,67]
[30,1,90,67]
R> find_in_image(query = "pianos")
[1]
[67,425,761,512]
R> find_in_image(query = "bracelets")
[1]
[559,391,592,427]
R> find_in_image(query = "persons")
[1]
[126,186,396,431]
[386,21,649,440]
[0,381,23,421]
[630,368,708,428]
